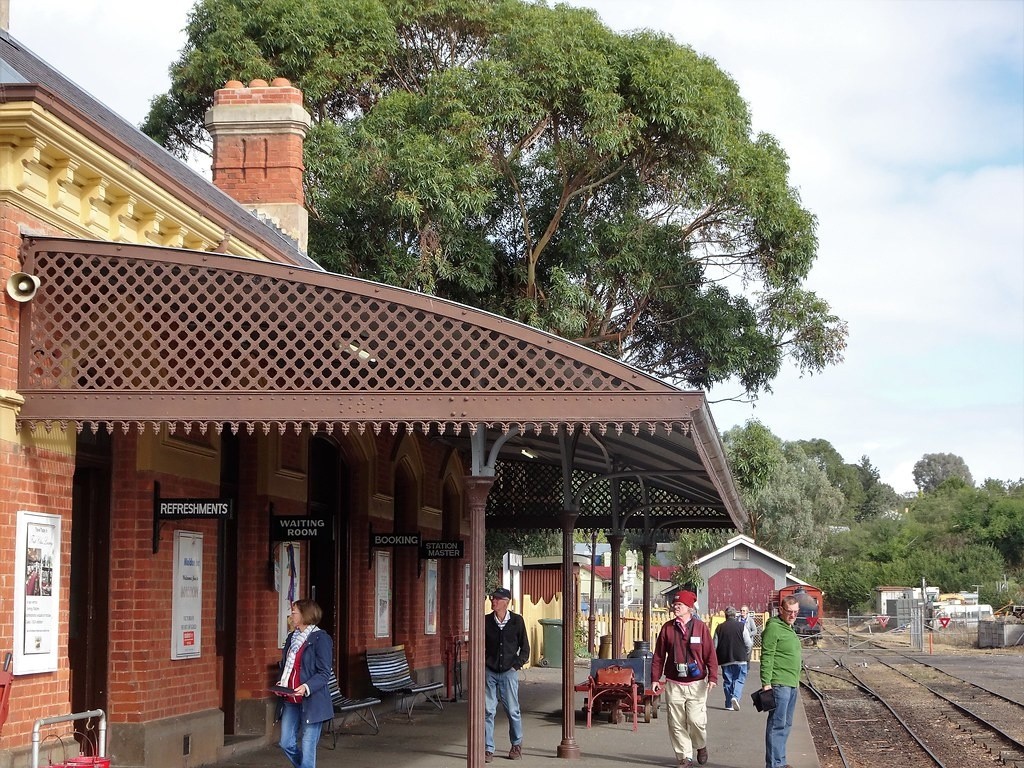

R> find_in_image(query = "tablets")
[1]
[759,689,776,711]
[269,685,297,695]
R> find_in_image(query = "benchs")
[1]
[365,643,445,721]
[273,661,381,750]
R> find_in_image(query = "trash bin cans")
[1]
[538,618,563,668]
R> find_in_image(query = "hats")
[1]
[490,588,512,599]
[672,590,697,609]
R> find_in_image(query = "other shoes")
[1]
[697,746,708,764]
[676,758,693,768]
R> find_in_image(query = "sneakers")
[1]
[485,751,493,763]
[730,695,740,711]
[509,745,522,760]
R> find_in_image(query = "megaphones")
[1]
[6,271,40,302]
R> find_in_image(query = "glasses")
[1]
[290,612,299,616]
[740,610,745,612]
[780,605,799,615]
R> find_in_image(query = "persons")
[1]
[274,599,335,768]
[712,605,752,712]
[759,596,802,768]
[650,591,719,768]
[738,605,757,672]
[485,588,531,762]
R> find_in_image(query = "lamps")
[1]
[335,339,378,364]
[520,447,539,460]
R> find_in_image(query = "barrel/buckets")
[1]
[38,724,111,768]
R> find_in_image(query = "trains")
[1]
[778,584,823,645]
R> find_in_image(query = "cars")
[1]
[581,592,590,610]
